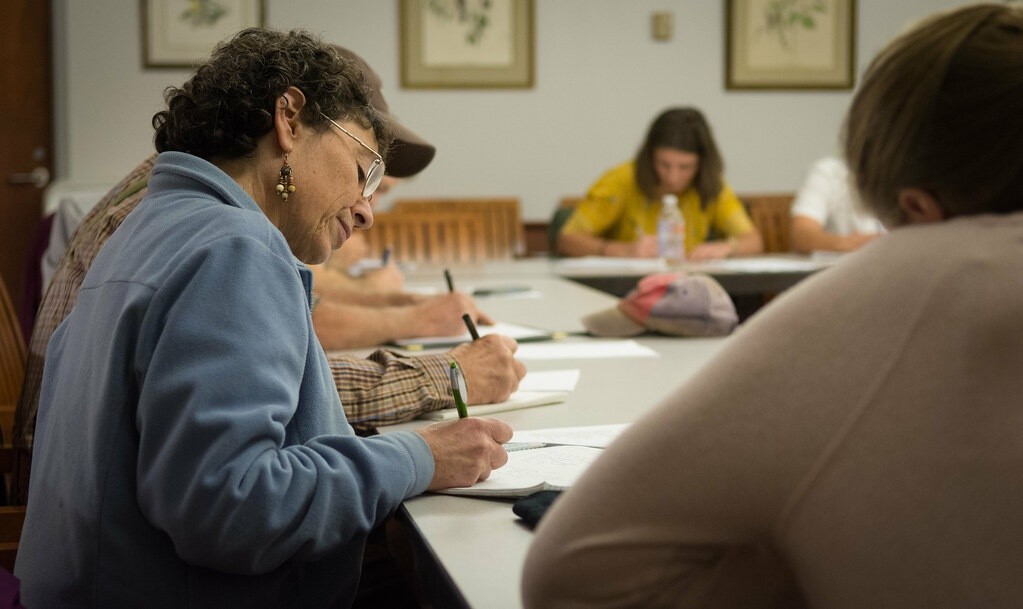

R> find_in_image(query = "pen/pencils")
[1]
[445,269,454,290]
[381,245,392,266]
[463,313,480,339]
[451,363,469,418]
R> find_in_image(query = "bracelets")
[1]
[601,240,608,254]
[726,236,737,259]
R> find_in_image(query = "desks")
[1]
[40,176,118,300]
[334,255,854,609]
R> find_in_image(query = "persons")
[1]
[556,105,767,267]
[15,26,516,609]
[15,151,528,504]
[784,159,888,258]
[519,1,1023,609]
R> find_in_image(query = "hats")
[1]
[583,270,738,340]
[324,43,437,178]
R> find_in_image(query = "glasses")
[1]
[318,104,385,204]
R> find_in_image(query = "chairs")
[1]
[741,192,796,254]
[395,197,529,256]
[0,269,32,501]
[364,207,481,259]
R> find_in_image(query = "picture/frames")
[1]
[400,0,534,89]
[722,0,858,91]
[140,0,269,69]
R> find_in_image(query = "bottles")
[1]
[655,194,687,267]
